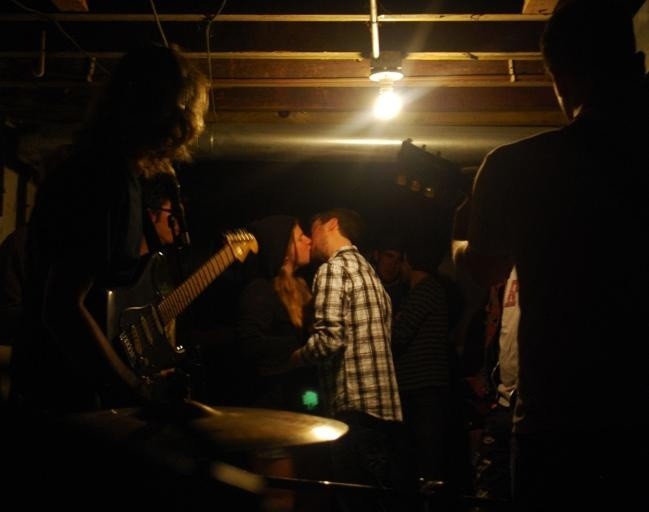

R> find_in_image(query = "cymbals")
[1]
[73,399,348,448]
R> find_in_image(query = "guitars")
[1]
[93,230,259,392]
[394,138,474,192]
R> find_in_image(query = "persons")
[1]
[1,206,520,511]
[448,2,649,512]
[0,41,214,509]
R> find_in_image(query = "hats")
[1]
[245,213,296,278]
